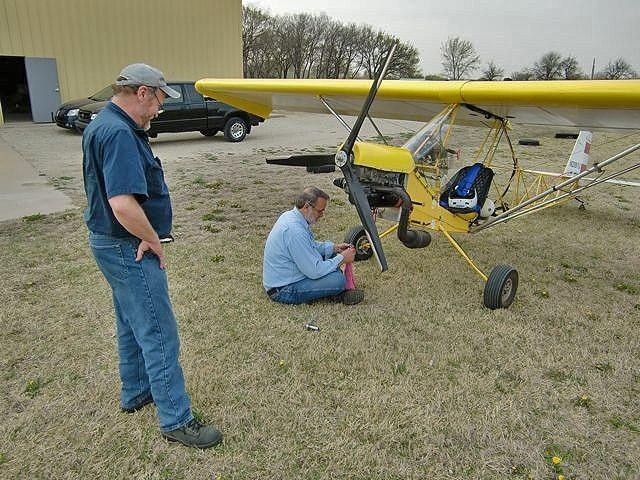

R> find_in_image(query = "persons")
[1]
[262,186,365,304]
[82,63,223,450]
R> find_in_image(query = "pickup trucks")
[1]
[75,81,264,142]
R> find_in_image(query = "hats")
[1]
[115,63,182,100]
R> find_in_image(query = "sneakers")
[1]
[316,289,365,304]
[160,418,222,448]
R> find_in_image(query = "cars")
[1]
[55,83,114,130]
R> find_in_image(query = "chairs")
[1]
[437,162,495,218]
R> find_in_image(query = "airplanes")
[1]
[194,40,640,309]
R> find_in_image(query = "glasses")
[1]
[149,86,166,116]
